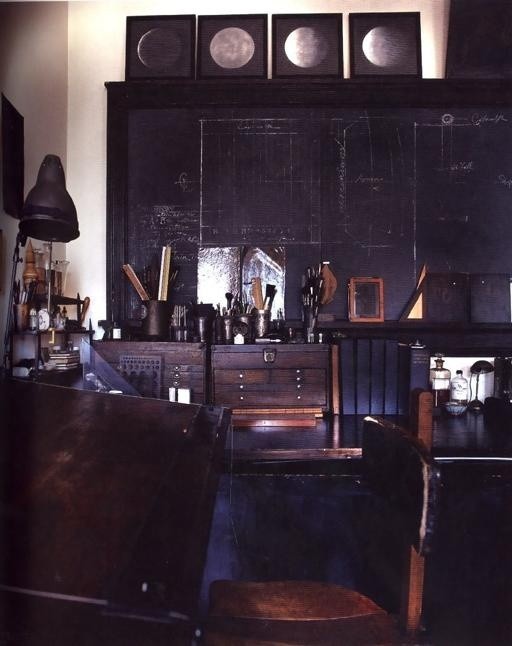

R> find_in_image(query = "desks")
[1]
[315,320,511,427]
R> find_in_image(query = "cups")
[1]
[113,329,121,340]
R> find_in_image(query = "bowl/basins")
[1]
[445,402,467,416]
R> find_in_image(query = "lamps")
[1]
[3,155,79,375]
[468,360,495,414]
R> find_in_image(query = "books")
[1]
[48,351,79,370]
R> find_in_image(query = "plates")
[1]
[38,310,49,331]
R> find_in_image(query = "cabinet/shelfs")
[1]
[10,295,330,453]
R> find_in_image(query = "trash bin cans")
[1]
[209,579,388,646]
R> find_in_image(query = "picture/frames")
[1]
[125,14,196,81]
[349,11,422,79]
[196,13,268,80]
[271,12,344,80]
[349,276,384,323]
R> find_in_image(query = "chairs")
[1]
[201,389,443,646]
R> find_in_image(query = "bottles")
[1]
[430,359,468,408]
